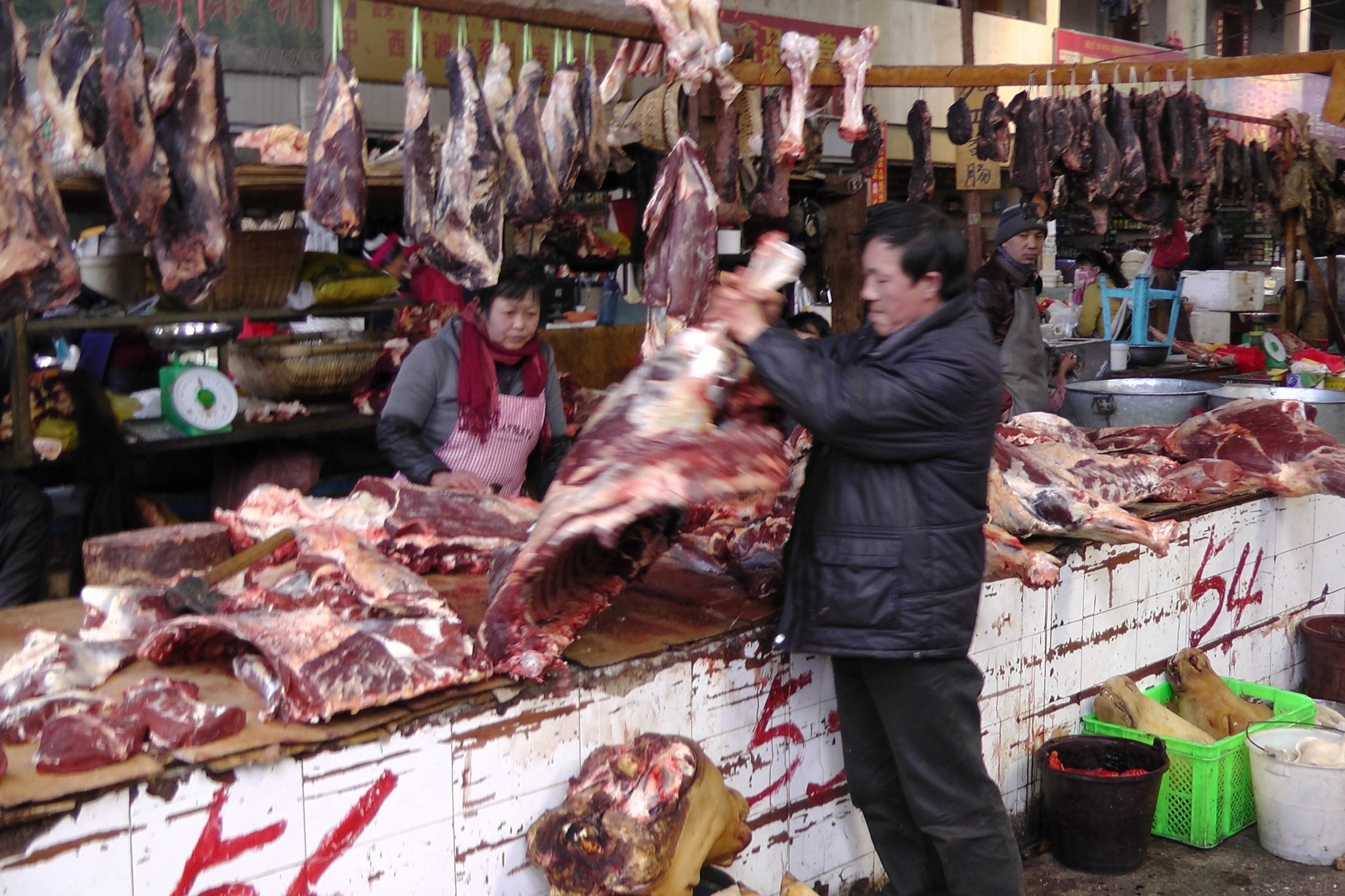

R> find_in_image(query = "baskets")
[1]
[227,331,387,404]
[1082,672,1321,843]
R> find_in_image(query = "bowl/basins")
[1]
[1207,382,1345,442]
[1126,344,1171,366]
[1064,378,1224,429]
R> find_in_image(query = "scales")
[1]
[148,322,238,434]
[1239,311,1290,369]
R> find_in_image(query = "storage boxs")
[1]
[1178,268,1265,313]
[1080,676,1320,852]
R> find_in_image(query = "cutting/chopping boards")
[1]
[83,521,230,582]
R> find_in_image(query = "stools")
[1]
[1096,273,1186,357]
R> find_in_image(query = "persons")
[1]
[376,254,568,493]
[1151,183,1224,291]
[976,204,1046,422]
[1046,352,1078,413]
[1072,249,1129,338]
[717,206,1025,896]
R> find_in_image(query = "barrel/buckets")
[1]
[1034,736,1171,875]
[1244,719,1345,867]
[1110,344,1130,370]
[79,250,147,304]
[1300,614,1345,705]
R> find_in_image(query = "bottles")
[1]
[597,280,620,325]
[1108,215,1142,229]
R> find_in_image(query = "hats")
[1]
[992,199,1047,246]
